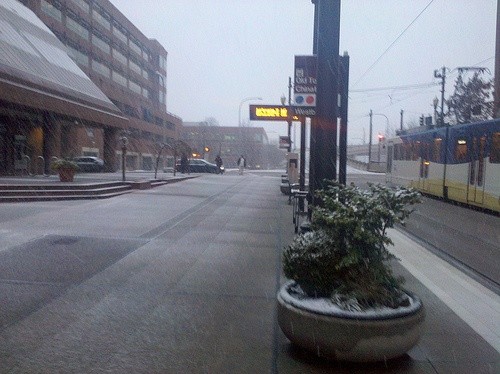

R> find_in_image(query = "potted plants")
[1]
[278,179,426,365]
[54,149,82,182]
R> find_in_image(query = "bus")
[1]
[386,118,500,214]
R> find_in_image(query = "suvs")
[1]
[175,158,225,174]
[73,156,104,172]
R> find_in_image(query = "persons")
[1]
[181,153,188,174]
[215,155,225,175]
[237,154,246,173]
